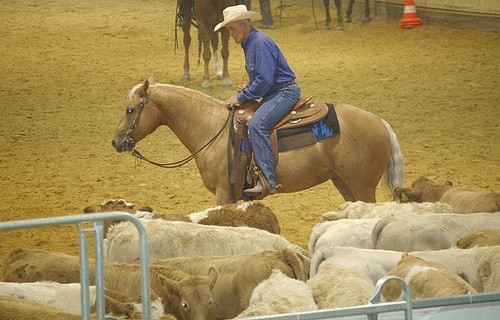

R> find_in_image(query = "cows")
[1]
[0,174,500,320]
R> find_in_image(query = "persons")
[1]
[222,5,301,196]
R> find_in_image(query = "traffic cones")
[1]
[400,0,422,29]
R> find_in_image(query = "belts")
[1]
[270,79,296,94]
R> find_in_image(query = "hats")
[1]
[214,4,263,32]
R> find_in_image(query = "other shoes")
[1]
[244,180,277,196]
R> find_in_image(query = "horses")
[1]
[111,74,405,206]
[324,0,374,29]
[174,0,253,89]
